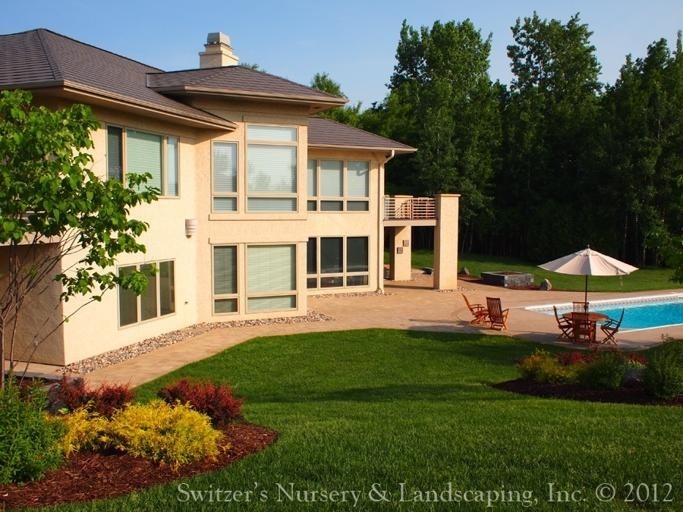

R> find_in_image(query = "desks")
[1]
[562,312,608,343]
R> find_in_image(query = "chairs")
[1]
[554,306,574,341]
[486,297,509,331]
[600,308,624,346]
[572,302,589,339]
[462,294,489,324]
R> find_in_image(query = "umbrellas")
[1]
[537,244,640,339]
